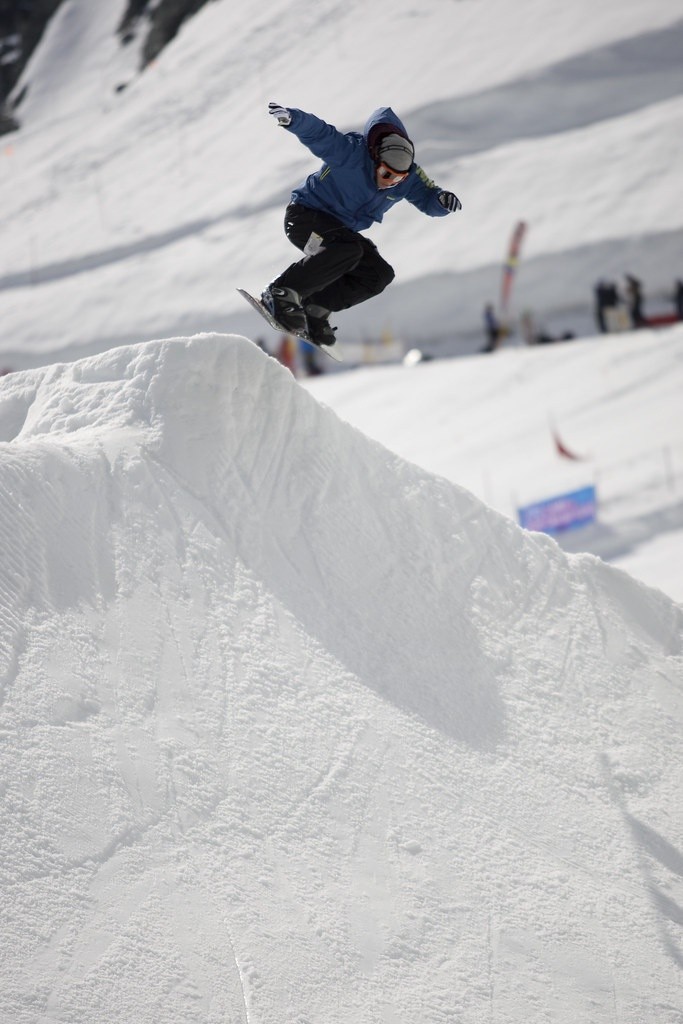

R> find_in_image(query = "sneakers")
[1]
[304,305,338,345]
[261,283,309,334]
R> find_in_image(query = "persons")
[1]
[480,271,683,352]
[255,336,323,378]
[258,99,464,347]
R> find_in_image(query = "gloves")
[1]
[268,102,292,127]
[439,193,462,212]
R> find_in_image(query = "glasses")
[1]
[376,163,408,184]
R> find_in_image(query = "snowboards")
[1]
[236,285,346,366]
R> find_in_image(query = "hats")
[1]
[378,133,414,176]
